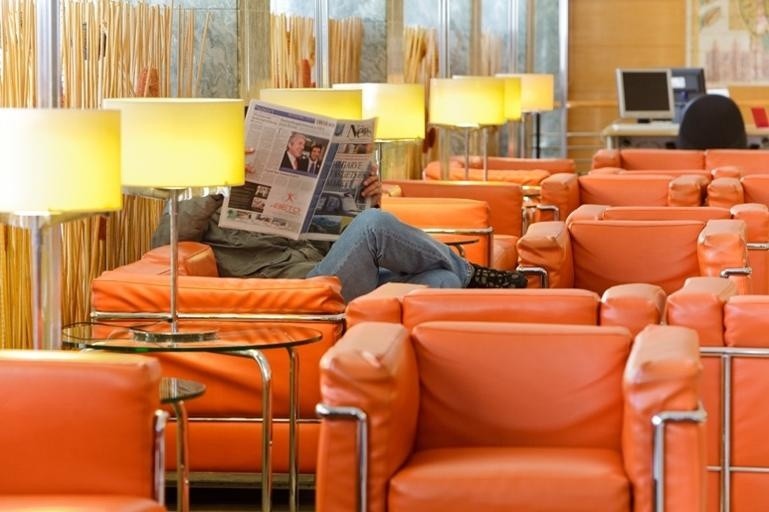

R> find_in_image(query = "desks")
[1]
[598,115,769,151]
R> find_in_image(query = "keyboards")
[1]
[613,122,680,134]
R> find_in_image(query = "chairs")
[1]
[339,148,767,326]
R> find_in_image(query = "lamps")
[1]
[262,88,361,120]
[428,79,480,179]
[333,83,426,184]
[106,98,246,341]
[3,107,124,350]
[478,74,554,183]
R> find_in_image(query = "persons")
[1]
[281,134,306,170]
[304,145,322,175]
[152,107,527,305]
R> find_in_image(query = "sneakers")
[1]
[468,261,528,288]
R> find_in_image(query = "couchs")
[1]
[86,237,344,489]
[2,354,165,509]
[665,289,769,512]
[313,321,707,512]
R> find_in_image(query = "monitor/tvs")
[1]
[616,67,675,124]
[672,67,706,123]
